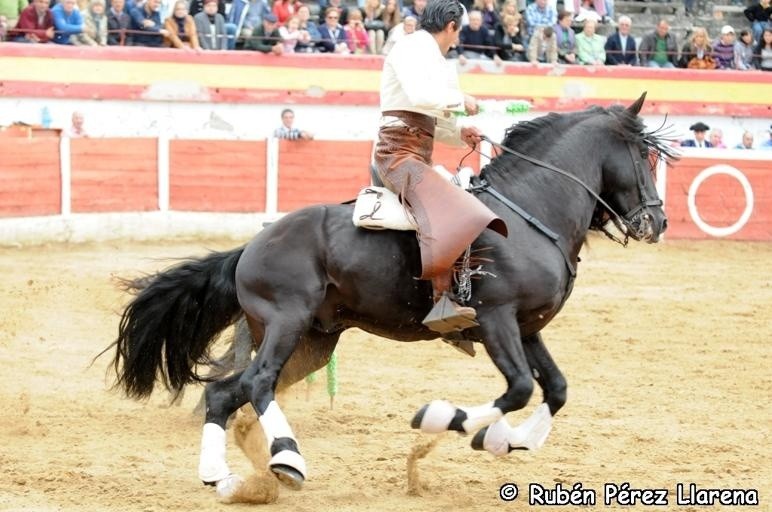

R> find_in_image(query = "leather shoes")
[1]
[449,340,475,355]
[428,293,478,333]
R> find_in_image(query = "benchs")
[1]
[614,1,770,44]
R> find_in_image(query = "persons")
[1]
[735,131,754,150]
[59,110,89,139]
[709,128,728,149]
[760,124,772,147]
[274,108,315,141]
[1,0,771,75]
[361,0,485,358]
[671,121,713,147]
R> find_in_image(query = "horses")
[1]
[88,91,684,506]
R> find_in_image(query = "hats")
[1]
[263,13,277,24]
[90,0,106,14]
[689,123,708,131]
[722,25,734,35]
